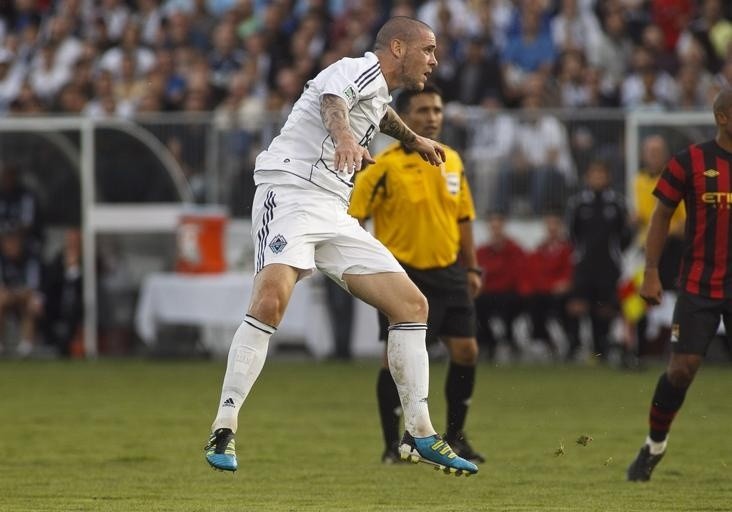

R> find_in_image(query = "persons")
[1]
[205,16,479,480]
[627,84,730,481]
[348,80,488,467]
[1,1,730,370]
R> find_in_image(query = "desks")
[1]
[133,269,340,361]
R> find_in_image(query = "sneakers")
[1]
[380,439,417,468]
[439,427,488,466]
[395,428,480,480]
[204,426,240,476]
[625,439,667,484]
